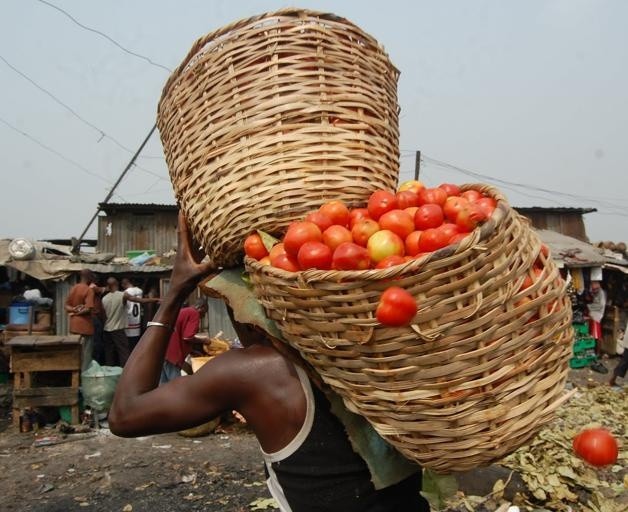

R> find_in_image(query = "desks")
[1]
[5,335,84,434]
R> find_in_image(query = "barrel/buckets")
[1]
[8,302,35,324]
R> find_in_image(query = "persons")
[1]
[609,324,628,387]
[159,298,209,387]
[585,281,606,356]
[107,208,432,512]
[64,268,164,372]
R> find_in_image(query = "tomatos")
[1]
[376,287,417,327]
[244,181,566,320]
[572,429,618,467]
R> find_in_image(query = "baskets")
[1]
[156,8,401,268]
[243,182,573,474]
